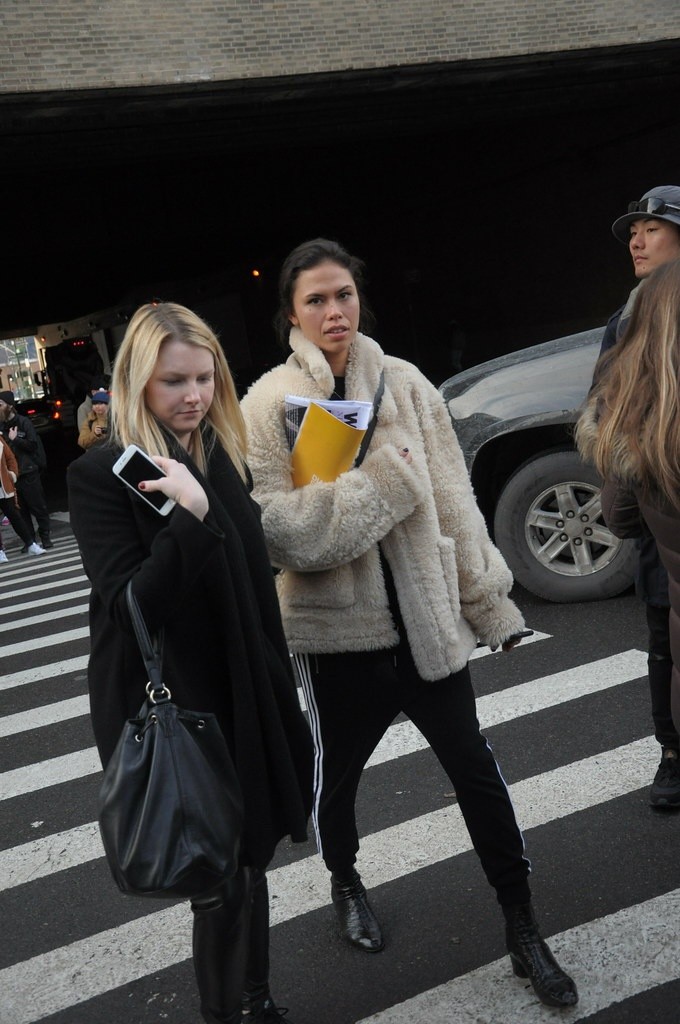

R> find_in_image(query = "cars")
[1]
[436,323,642,604]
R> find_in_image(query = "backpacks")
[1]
[16,415,47,469]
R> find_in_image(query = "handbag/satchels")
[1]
[93,578,245,902]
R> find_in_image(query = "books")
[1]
[285,394,372,488]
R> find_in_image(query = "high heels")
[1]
[331,872,387,954]
[503,898,578,1008]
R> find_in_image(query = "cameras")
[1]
[100,427,107,434]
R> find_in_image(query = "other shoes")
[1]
[40,532,53,548]
[21,539,37,553]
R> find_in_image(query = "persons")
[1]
[77,379,111,453]
[64,301,315,1024]
[574,184,679,808]
[0,435,47,564]
[237,238,580,1009]
[0,391,53,553]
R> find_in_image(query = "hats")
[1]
[611,185,680,246]
[90,391,110,404]
[0,390,15,407]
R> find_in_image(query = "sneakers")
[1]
[0,550,9,563]
[1,517,10,526]
[238,990,294,1024]
[28,542,46,556]
[649,743,679,810]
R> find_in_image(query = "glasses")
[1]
[627,197,680,218]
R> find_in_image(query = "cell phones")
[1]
[112,444,177,516]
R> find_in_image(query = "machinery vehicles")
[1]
[12,334,113,437]
